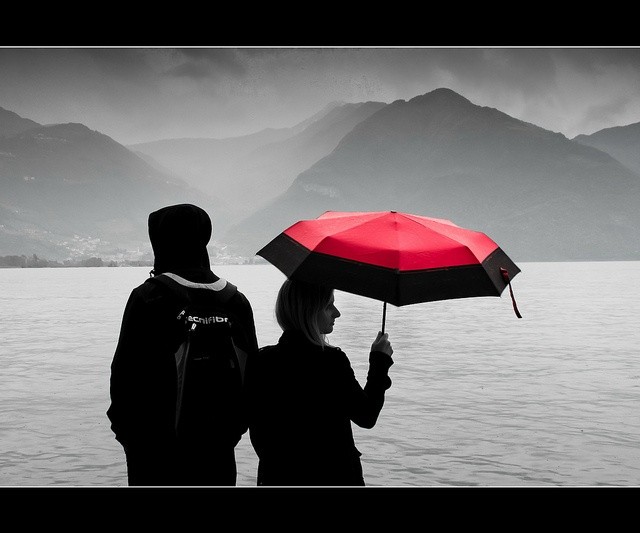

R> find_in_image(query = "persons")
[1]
[249,278,393,487]
[106,203,260,487]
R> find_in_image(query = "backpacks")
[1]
[154,275,254,451]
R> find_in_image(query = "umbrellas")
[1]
[256,210,521,335]
